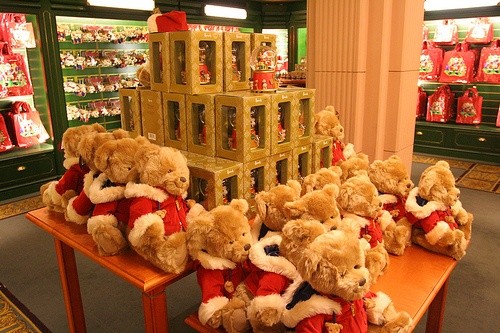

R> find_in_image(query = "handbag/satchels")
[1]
[415,17,500,128]
[0,13,50,154]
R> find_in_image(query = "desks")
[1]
[182,245,458,333]
[27,204,193,333]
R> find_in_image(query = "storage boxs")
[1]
[120,32,334,209]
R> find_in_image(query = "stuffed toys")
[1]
[56,24,150,122]
[154,42,306,212]
[136,7,189,87]
[314,105,370,166]
[188,151,474,333]
[40,122,210,274]
